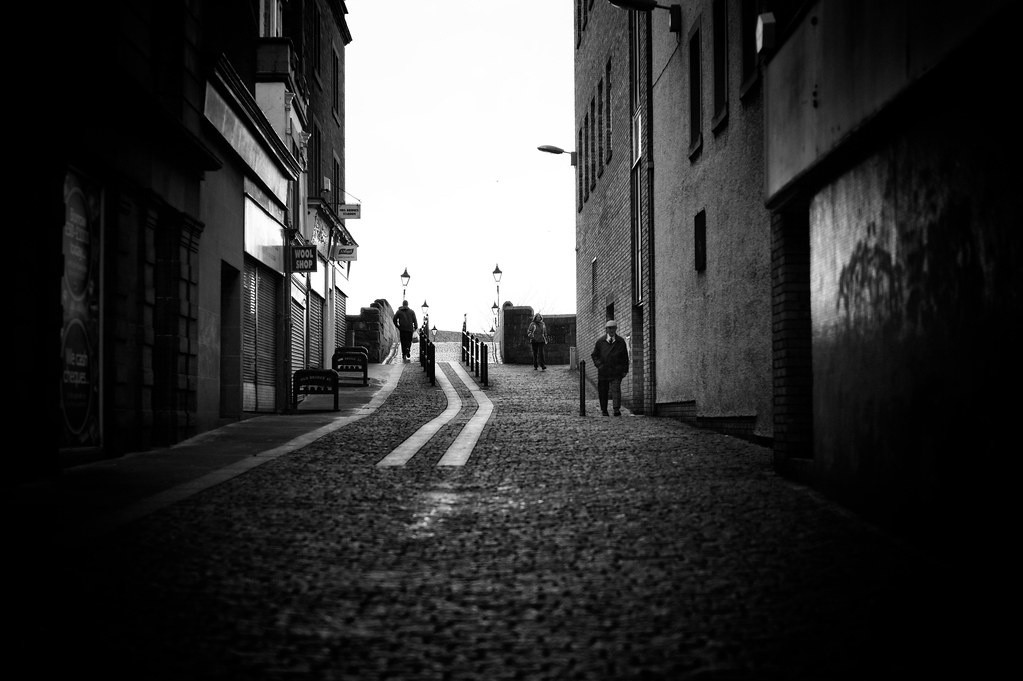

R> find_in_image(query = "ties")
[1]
[609,337,612,344]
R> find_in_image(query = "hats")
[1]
[605,320,617,328]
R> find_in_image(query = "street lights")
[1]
[421,299,430,320]
[400,267,410,302]
[492,302,500,327]
[431,325,438,342]
[493,263,502,328]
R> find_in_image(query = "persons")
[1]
[591,320,629,416]
[393,300,418,360]
[527,313,548,370]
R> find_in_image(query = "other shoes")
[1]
[602,411,609,417]
[534,367,537,370]
[614,410,621,416]
[542,366,546,370]
